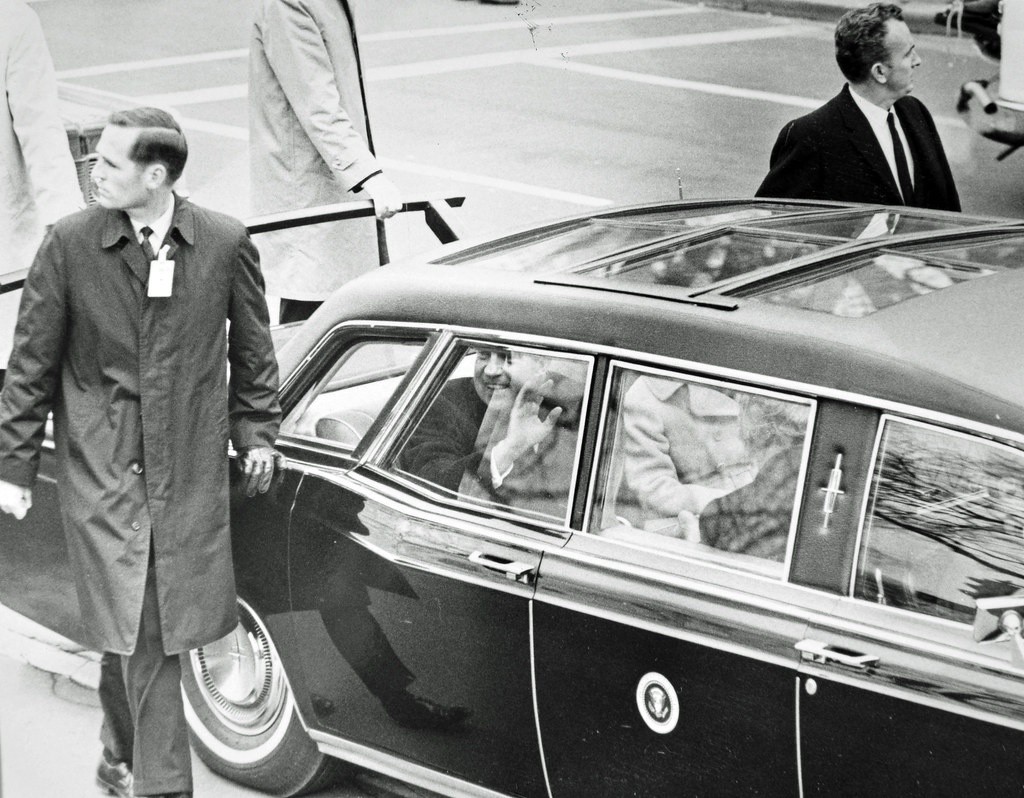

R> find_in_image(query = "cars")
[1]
[0,191,1024,797]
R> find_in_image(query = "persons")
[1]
[620,374,767,548]
[247,1,405,334]
[227,459,473,738]
[394,347,586,522]
[0,0,87,380]
[0,107,286,797]
[755,2,963,213]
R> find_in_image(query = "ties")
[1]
[139,227,154,262]
[886,108,916,207]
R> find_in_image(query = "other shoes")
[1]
[398,697,470,730]
[309,692,332,715]
[95,752,135,798]
